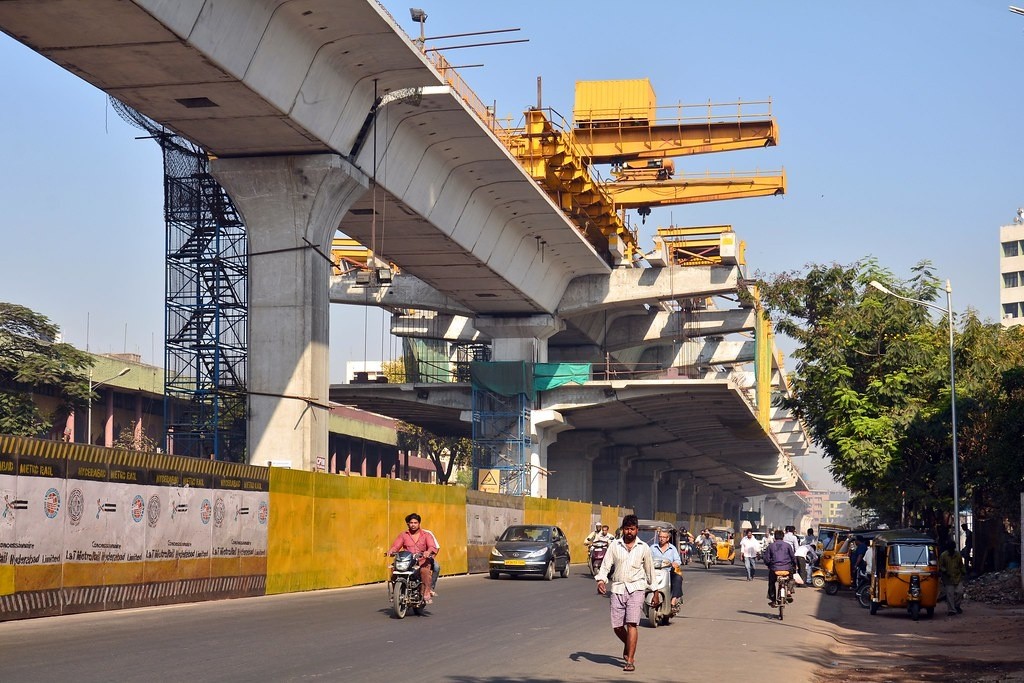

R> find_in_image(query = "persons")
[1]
[387,514,441,605]
[740,529,761,582]
[648,531,683,611]
[584,522,615,569]
[763,531,794,607]
[850,536,873,587]
[824,532,834,550]
[938,540,963,614]
[594,514,659,671]
[784,525,817,587]
[679,525,717,563]
[960,524,974,566]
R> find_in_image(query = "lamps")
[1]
[417,390,429,400]
[355,269,392,285]
[603,388,615,398]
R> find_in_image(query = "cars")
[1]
[488,524,571,582]
[637,519,681,559]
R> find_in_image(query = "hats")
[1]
[596,522,601,526]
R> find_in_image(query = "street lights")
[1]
[871,278,962,554]
[88,367,132,446]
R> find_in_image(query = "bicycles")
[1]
[768,566,797,621]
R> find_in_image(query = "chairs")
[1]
[542,531,549,540]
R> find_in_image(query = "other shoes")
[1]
[948,610,956,616]
[956,608,963,614]
[768,602,776,608]
[430,590,438,597]
[787,593,793,603]
[425,597,433,604]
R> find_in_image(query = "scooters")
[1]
[639,556,684,628]
[585,536,614,579]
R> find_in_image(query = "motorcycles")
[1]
[747,521,927,608]
[680,537,718,570]
[708,525,736,565]
[868,528,941,621]
[383,550,433,619]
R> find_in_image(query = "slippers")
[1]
[624,663,635,671]
[671,604,677,611]
[623,645,626,660]
